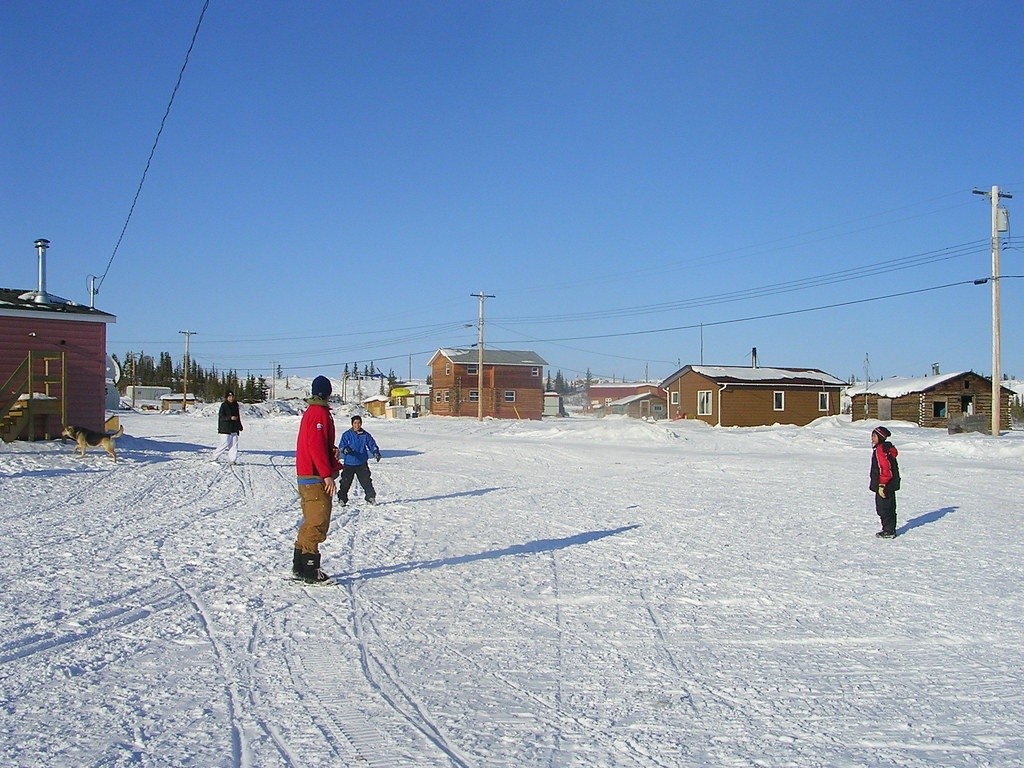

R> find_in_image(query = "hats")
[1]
[224,390,234,398]
[351,415,363,425]
[871,426,892,441]
[312,375,332,397]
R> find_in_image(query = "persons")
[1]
[214,391,243,464]
[869,427,900,539]
[338,416,381,507]
[292,375,343,586]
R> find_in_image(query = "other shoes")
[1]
[364,498,377,506]
[338,497,346,507]
[876,531,896,539]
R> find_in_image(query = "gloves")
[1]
[372,450,381,462]
[343,447,351,455]
[877,484,887,498]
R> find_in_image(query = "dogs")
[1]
[60,425,124,463]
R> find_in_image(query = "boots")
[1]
[292,546,306,581]
[300,553,333,586]
